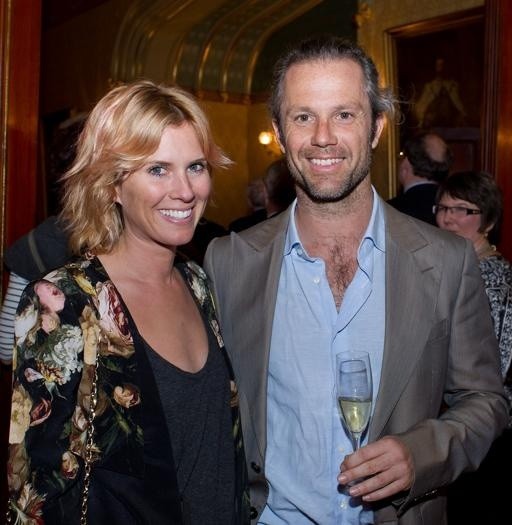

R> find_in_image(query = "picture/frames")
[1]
[380,3,498,207]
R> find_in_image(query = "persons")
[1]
[424,168,512,376]
[226,175,268,233]
[206,33,509,524]
[179,202,227,260]
[257,156,297,221]
[399,45,469,135]
[2,127,95,385]
[382,131,452,227]
[3,77,252,523]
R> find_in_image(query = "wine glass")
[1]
[334,348,375,489]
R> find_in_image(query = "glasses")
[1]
[430,204,484,217]
